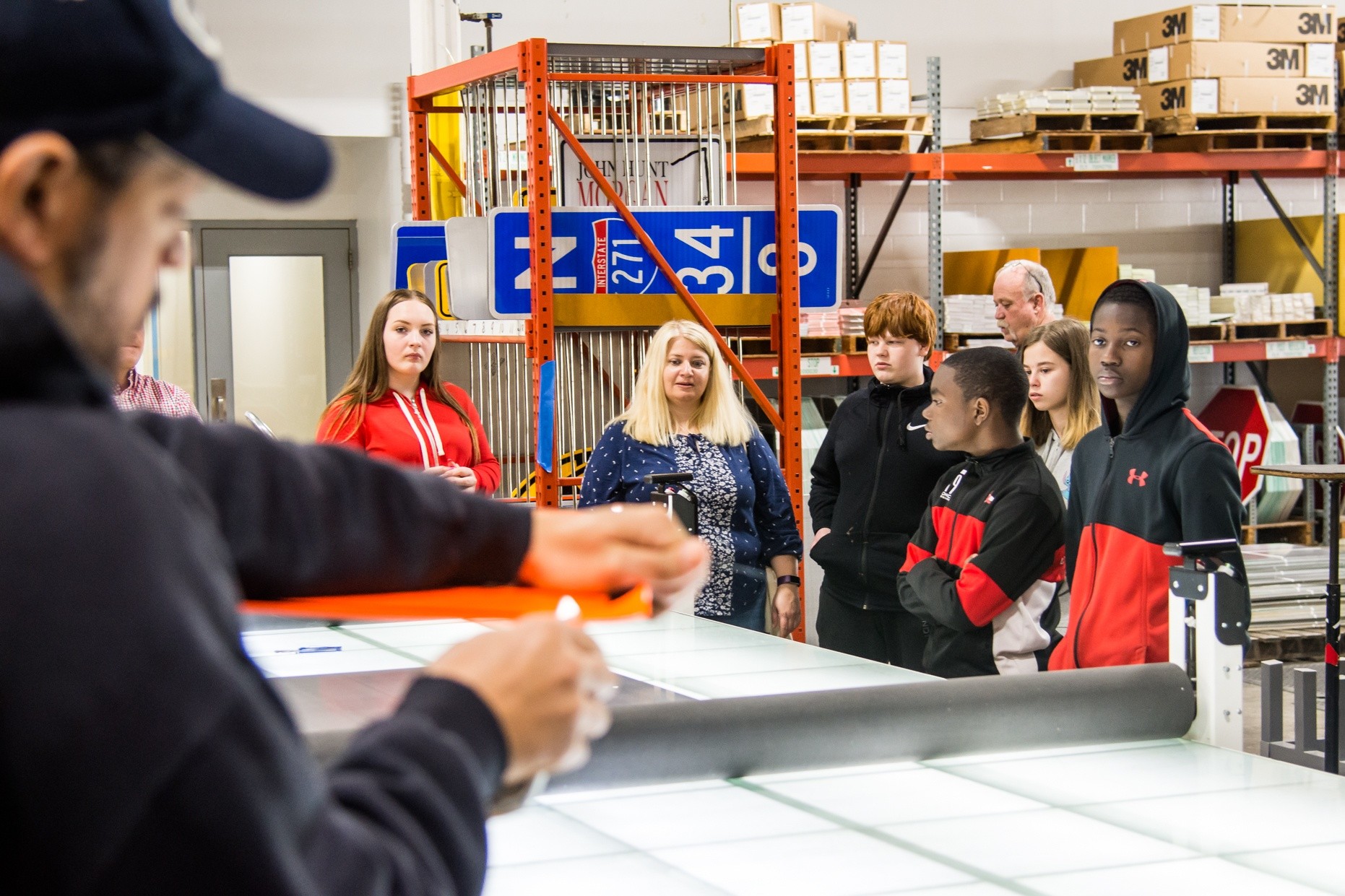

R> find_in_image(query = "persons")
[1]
[577,319,805,638]
[314,289,502,498]
[807,292,968,671]
[1048,279,1251,671]
[0,0,716,896]
[895,346,1068,679]
[992,260,1102,638]
[110,322,205,425]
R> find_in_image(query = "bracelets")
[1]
[777,575,801,587]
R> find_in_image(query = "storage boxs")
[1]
[560,0,1345,551]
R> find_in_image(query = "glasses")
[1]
[1005,259,1043,295]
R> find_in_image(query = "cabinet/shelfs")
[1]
[401,35,815,641]
[704,137,1345,562]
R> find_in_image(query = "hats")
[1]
[0,0,332,202]
